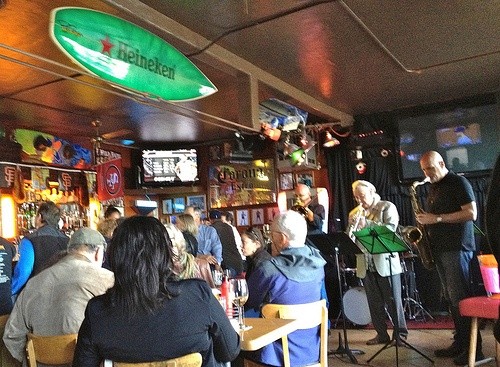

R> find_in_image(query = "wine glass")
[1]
[229,278,252,331]
[211,268,233,287]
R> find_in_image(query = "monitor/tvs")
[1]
[392,93,500,184]
[135,143,200,187]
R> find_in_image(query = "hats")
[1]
[210,209,222,219]
[69,228,107,248]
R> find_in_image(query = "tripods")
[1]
[307,232,365,364]
[353,225,434,366]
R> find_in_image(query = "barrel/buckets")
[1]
[477,254,500,299]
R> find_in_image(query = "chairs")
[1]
[244,299,328,366]
[25,332,78,367]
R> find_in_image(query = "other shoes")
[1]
[366,333,391,345]
[393,328,408,346]
[435,342,465,356]
[454,347,484,365]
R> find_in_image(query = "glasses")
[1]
[264,230,289,238]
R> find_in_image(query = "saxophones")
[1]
[403,177,433,269]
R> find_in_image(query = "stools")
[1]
[459,293,500,367]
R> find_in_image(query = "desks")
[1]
[230,317,298,351]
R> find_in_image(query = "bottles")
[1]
[16,199,82,235]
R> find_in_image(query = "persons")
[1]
[208,207,243,274]
[1,227,115,367]
[242,209,326,367]
[97,205,125,241]
[0,234,18,317]
[71,214,241,367]
[218,211,247,257]
[159,221,218,293]
[9,200,70,297]
[170,212,198,253]
[452,127,472,145]
[291,183,324,238]
[345,180,408,349]
[411,151,486,366]
[239,228,275,278]
[183,204,225,273]
